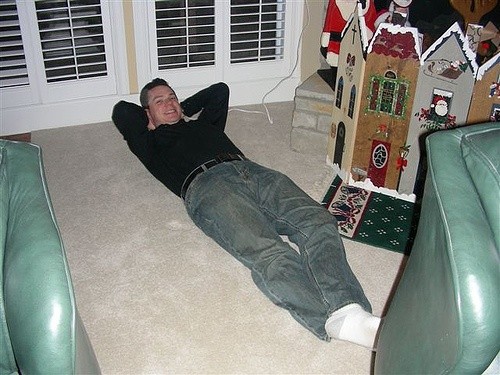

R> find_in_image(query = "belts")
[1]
[182,152,246,200]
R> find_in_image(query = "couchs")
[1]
[373,124,500,375]
[0,138,102,375]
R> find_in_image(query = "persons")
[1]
[112,78,383,352]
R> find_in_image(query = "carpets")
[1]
[307,166,420,256]
